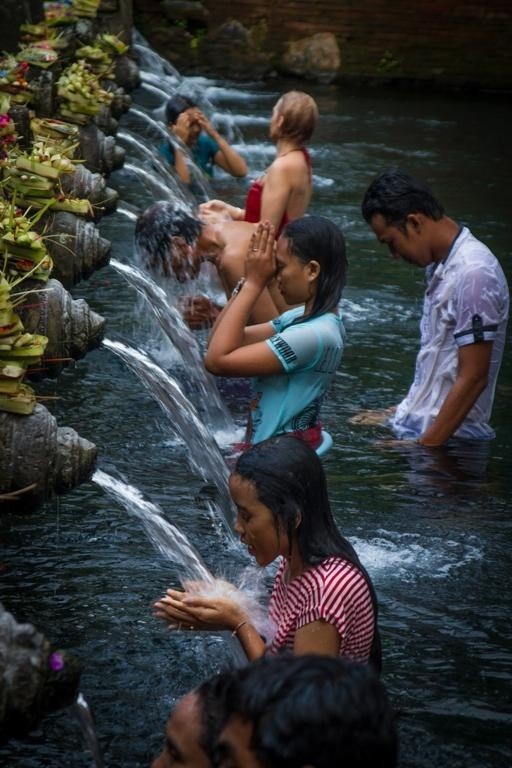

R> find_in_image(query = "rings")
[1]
[189,625,195,630]
[251,248,259,253]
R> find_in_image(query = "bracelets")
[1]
[237,205,244,220]
[231,279,243,298]
[229,618,252,637]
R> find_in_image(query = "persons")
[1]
[145,670,245,768]
[347,170,512,449]
[155,435,382,680]
[199,90,321,240]
[135,199,307,330]
[151,93,248,205]
[217,651,399,767]
[203,213,350,460]
